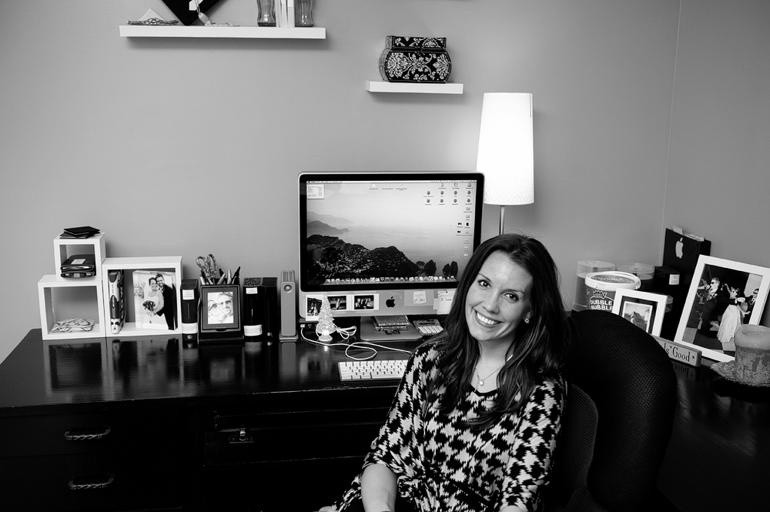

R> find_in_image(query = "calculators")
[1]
[412,319,444,336]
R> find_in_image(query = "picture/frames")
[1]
[202,283,239,329]
[611,288,667,345]
[676,254,770,369]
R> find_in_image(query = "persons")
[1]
[692,275,759,358]
[310,297,374,316]
[142,273,178,330]
[207,292,234,323]
[317,234,572,512]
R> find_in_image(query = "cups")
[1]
[733,324,769,384]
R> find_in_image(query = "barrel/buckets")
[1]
[585,269,640,311]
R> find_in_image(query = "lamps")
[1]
[475,90,534,235]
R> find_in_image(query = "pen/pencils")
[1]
[199,266,241,285]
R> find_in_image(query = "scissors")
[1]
[196,254,218,285]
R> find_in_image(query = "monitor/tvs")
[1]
[297,170,484,342]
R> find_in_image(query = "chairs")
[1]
[467,309,682,512]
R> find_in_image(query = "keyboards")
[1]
[336,359,409,381]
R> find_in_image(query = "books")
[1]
[108,270,127,334]
[60,225,100,278]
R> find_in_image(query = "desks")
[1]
[0,312,767,512]
